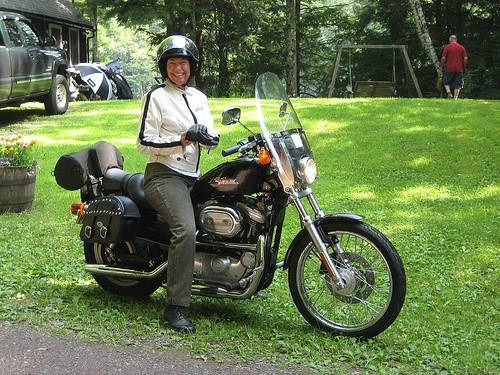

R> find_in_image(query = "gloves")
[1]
[185,129,218,146]
[190,124,208,133]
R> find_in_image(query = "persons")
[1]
[69,68,88,102]
[441,35,468,99]
[136,35,220,335]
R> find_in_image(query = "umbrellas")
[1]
[74,62,132,100]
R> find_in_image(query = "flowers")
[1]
[0,130,42,176]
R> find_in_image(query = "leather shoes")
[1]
[161,303,196,335]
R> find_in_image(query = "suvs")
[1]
[0,10,70,112]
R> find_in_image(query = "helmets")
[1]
[156,34,200,82]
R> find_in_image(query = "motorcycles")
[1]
[49,70,407,339]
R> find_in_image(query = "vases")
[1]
[0,165,37,215]
